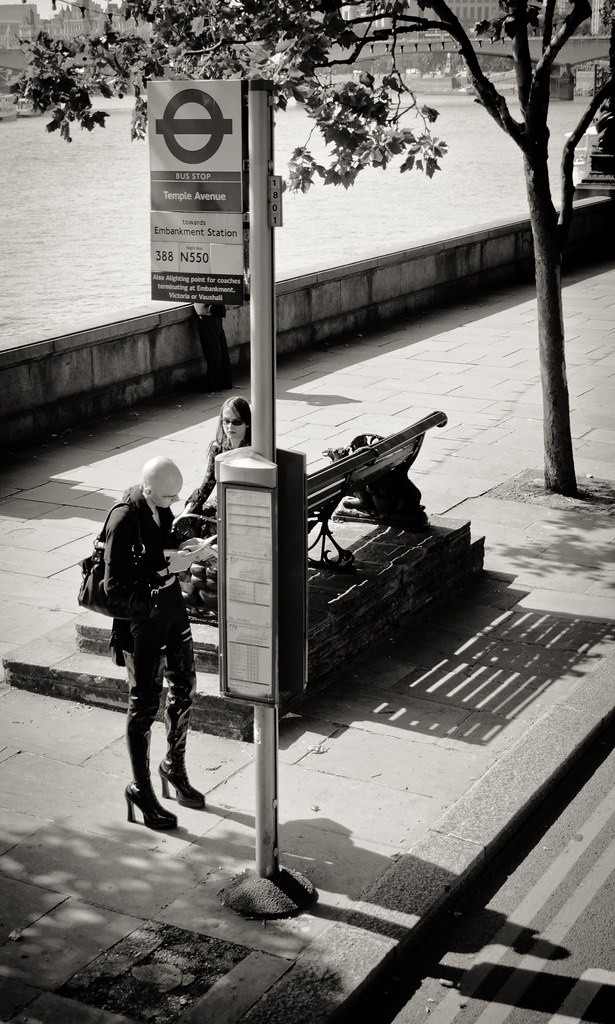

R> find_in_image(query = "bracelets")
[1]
[164,566,171,580]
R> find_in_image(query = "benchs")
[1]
[169,410,448,618]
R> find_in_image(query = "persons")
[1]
[103,456,205,831]
[173,396,252,532]
[192,303,233,392]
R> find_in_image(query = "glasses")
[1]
[220,416,246,425]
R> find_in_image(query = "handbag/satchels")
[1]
[78,503,153,620]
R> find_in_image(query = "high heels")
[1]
[125,781,177,829]
[158,758,207,809]
